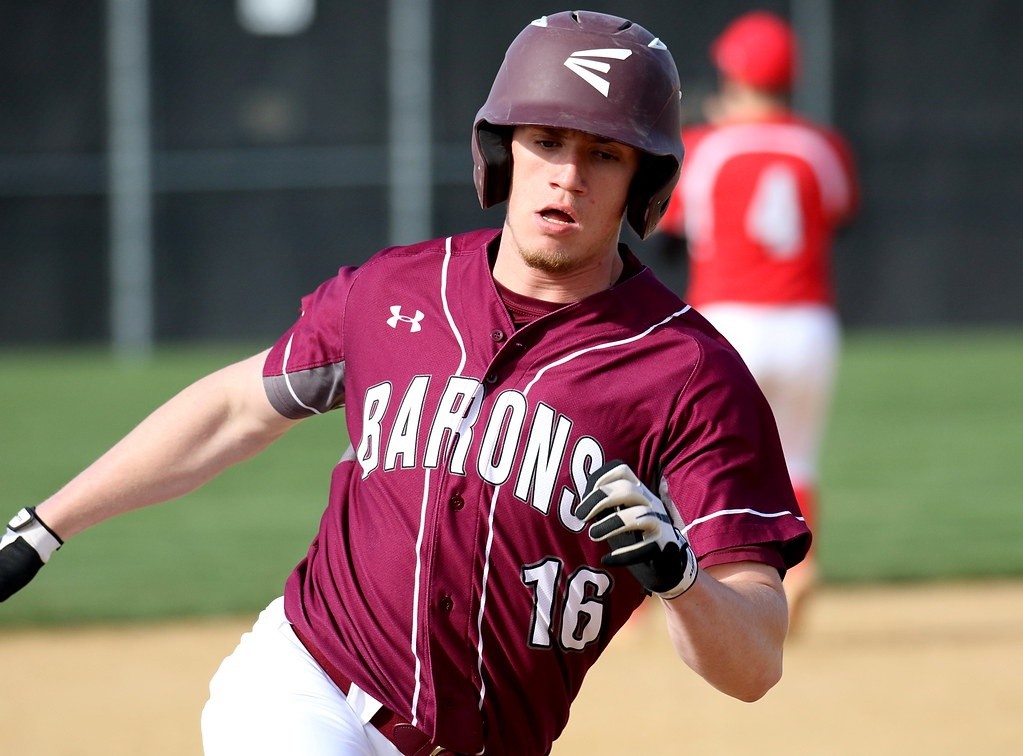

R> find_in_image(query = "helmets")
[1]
[471,10,684,242]
[711,12,799,93]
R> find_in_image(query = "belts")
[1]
[291,625,458,756]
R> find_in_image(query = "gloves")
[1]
[0,508,63,604]
[575,460,698,600]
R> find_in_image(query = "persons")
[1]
[0,11,811,756]
[640,9,858,639]
